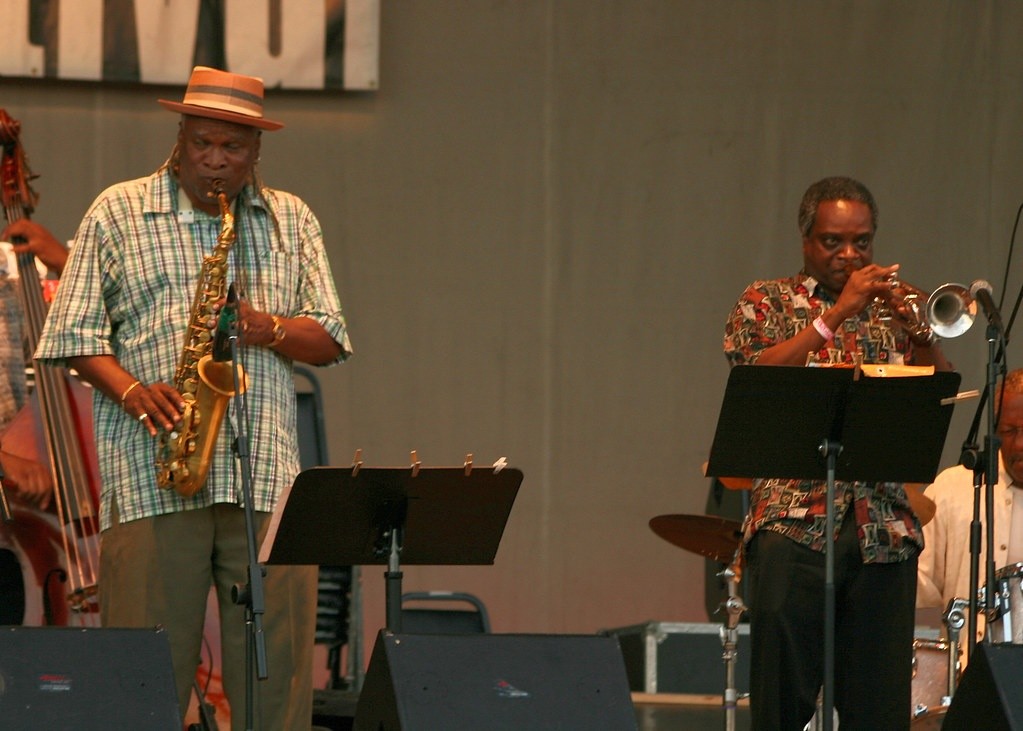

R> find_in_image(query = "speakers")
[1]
[0,624,182,731]
[939,640,1023,731]
[351,628,639,731]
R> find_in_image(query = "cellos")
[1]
[1,105,235,730]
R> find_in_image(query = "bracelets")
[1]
[121,381,141,408]
[812,316,835,340]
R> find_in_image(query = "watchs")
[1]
[267,315,286,347]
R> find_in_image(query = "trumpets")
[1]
[860,267,978,348]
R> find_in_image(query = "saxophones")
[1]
[151,172,252,501]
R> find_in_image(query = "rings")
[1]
[244,322,248,330]
[138,414,148,421]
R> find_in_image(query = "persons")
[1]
[32,65,354,731]
[0,218,69,512]
[915,368,1023,671]
[721,175,955,731]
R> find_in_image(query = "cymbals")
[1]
[648,511,753,567]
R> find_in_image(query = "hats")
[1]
[156,65,285,131]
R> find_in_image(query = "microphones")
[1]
[970,280,1005,332]
[213,282,239,362]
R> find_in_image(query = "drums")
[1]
[980,560,1022,646]
[908,635,965,731]
